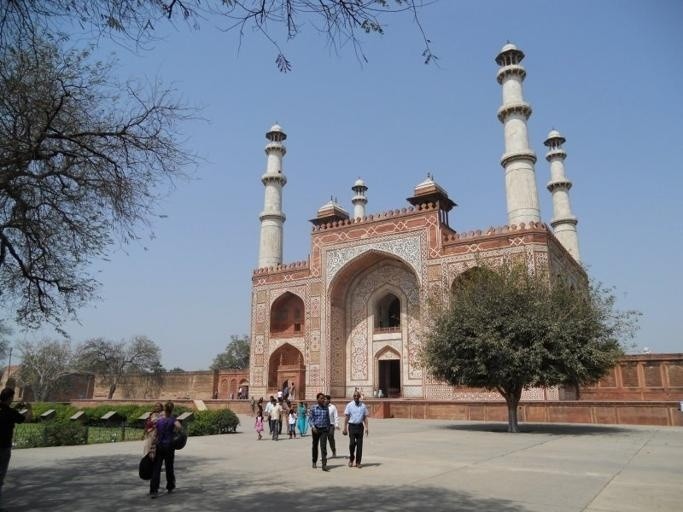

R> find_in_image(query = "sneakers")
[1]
[151,491,158,498]
[313,453,361,471]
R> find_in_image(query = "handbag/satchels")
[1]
[139,451,153,480]
[172,427,187,450]
[146,417,153,428]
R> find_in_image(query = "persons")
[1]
[318,396,340,462]
[0,384,32,488]
[229,392,234,400]
[377,388,384,400]
[306,392,329,471]
[141,401,160,459]
[213,389,217,399]
[341,390,369,468]
[371,388,377,399]
[236,385,242,399]
[135,402,185,498]
[247,380,310,441]
